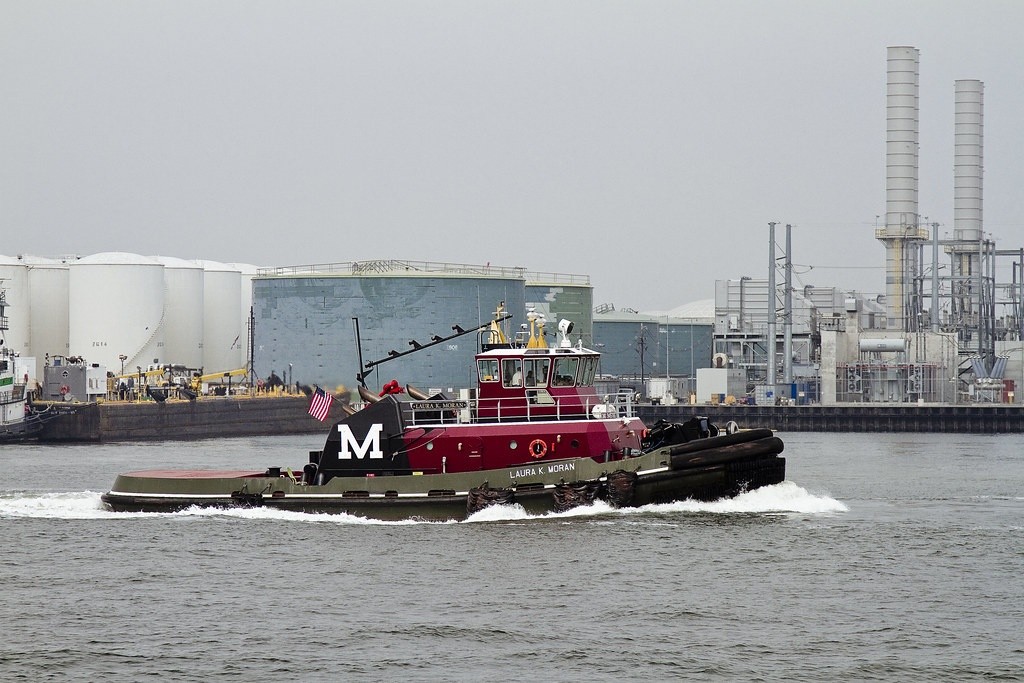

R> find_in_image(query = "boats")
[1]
[0,278,79,443]
[101,285,786,522]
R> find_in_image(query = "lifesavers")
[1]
[61,386,67,395]
[643,428,648,438]
[529,439,547,458]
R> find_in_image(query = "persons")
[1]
[511,367,522,387]
[525,370,535,387]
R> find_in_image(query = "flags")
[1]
[307,387,333,424]
